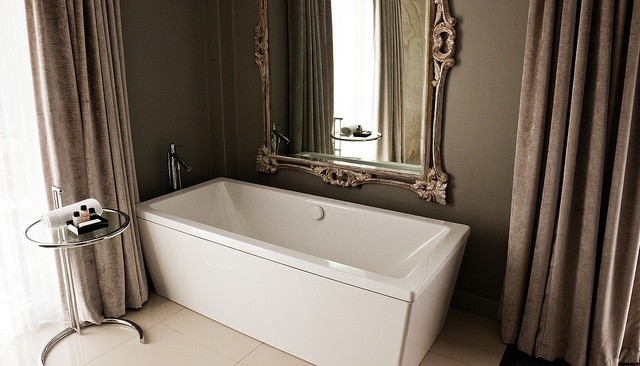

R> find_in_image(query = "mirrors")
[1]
[254,0,456,206]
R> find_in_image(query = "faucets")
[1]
[271,122,290,155]
[167,143,191,189]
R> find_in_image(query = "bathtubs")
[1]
[292,152,422,174]
[135,176,471,366]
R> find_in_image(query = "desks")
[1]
[26,185,145,366]
[331,117,381,158]
